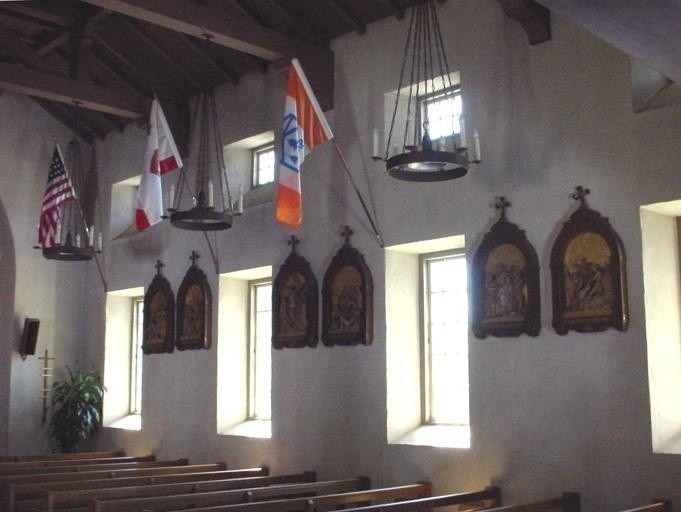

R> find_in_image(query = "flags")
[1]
[135,96,184,231]
[38,143,76,249]
[276,57,333,228]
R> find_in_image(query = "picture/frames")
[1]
[272,224,372,349]
[469,186,630,333]
[141,249,210,355]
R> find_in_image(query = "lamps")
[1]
[168,33,235,232]
[30,98,104,262]
[364,1,486,182]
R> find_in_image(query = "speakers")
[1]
[20,318,40,355]
